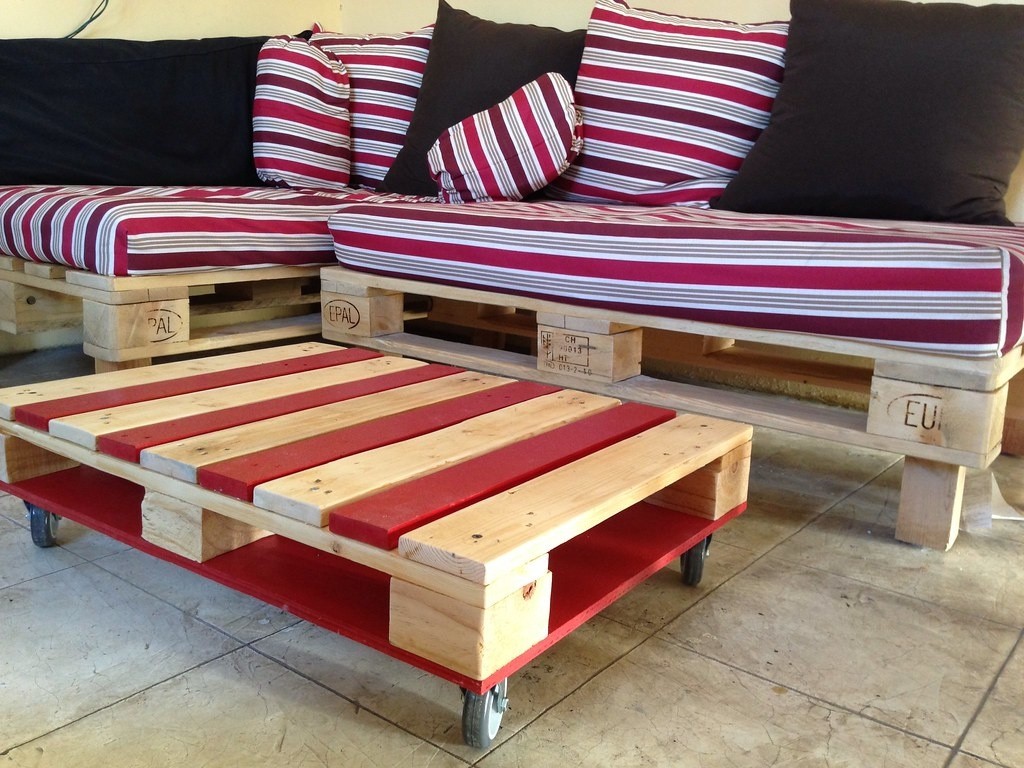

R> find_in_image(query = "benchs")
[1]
[0,186,442,376]
[319,199,1024,552]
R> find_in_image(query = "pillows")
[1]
[546,0,791,210]
[306,21,437,190]
[426,71,584,205]
[252,35,351,192]
[374,0,587,198]
[709,0,1024,227]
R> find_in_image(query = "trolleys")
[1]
[0,335,749,751]
